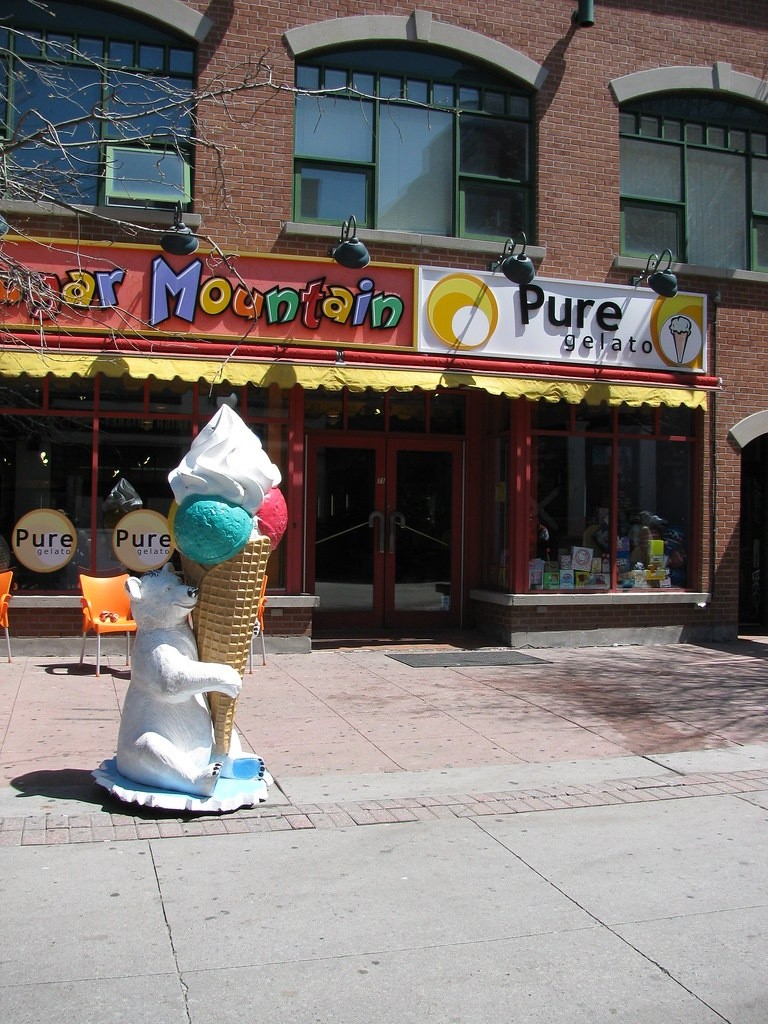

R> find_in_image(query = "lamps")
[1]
[160,199,199,256]
[0,214,9,238]
[632,249,678,298]
[490,231,535,284]
[328,214,371,269]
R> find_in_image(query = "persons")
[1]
[537,514,549,540]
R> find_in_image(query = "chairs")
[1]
[77,565,122,591]
[77,574,137,677]
[245,575,269,675]
[0,570,14,663]
